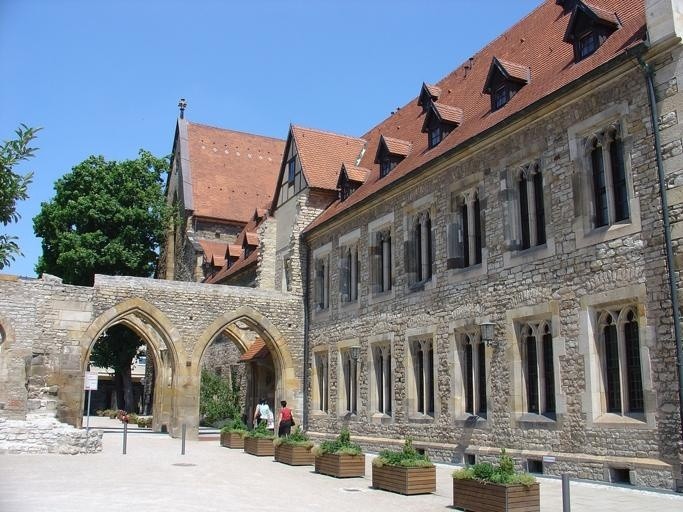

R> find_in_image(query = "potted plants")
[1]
[448,443,541,512]
[369,435,437,497]
[309,424,366,478]
[93,408,152,428]
[242,419,276,457]
[217,411,248,449]
[270,421,315,466]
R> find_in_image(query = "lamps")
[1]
[477,313,501,350]
[347,343,365,364]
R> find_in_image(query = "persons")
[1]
[278,401,297,437]
[254,399,269,428]
[267,401,274,431]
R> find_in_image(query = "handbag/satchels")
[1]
[256,407,262,419]
[290,411,295,427]
